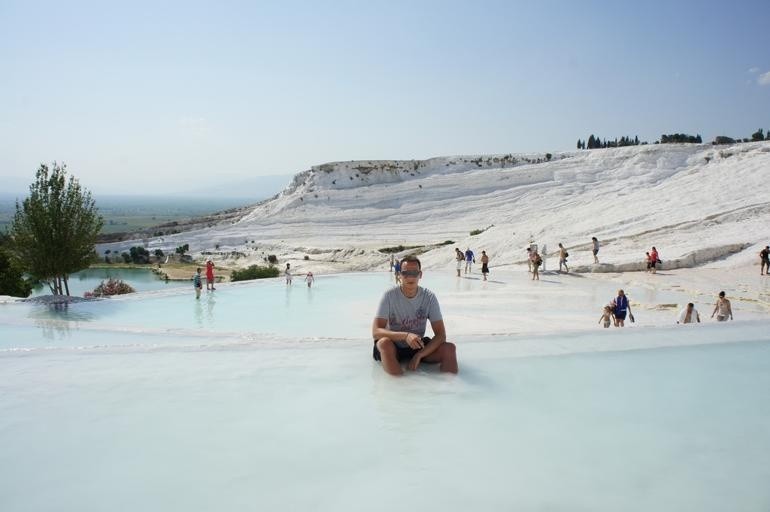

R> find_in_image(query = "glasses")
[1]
[400,270,421,278]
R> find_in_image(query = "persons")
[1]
[455,247,465,277]
[598,305,616,328]
[558,243,570,274]
[709,290,733,322]
[371,256,459,377]
[650,246,660,274]
[284,262,294,285]
[526,247,535,272]
[759,245,770,275]
[205,255,216,292]
[464,247,476,274]
[304,271,315,288]
[610,288,632,328]
[644,252,652,273]
[393,259,403,286]
[531,251,544,281]
[480,250,490,282]
[591,236,600,264]
[676,302,701,324]
[388,249,395,272]
[192,267,204,300]
